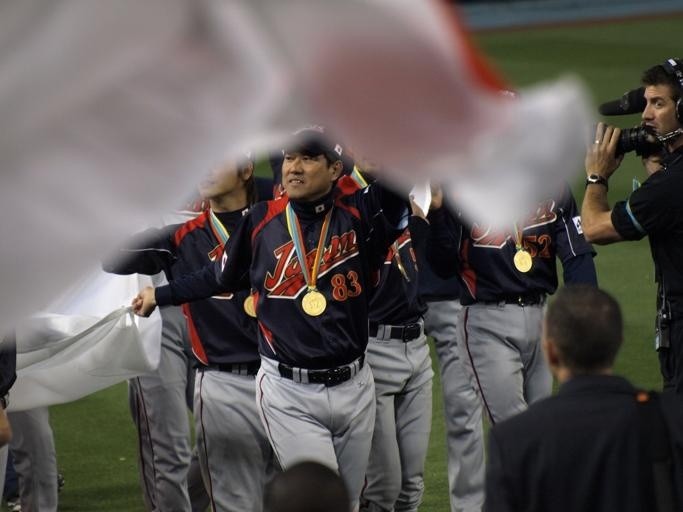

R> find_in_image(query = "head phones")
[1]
[662,58,683,124]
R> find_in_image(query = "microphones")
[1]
[646,128,683,142]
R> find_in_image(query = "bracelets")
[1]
[586,171,609,191]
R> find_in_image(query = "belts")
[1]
[279,353,365,386]
[506,294,539,304]
[368,324,420,339]
[196,361,260,371]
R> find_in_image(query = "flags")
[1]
[0,1,603,410]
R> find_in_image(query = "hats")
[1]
[287,128,353,176]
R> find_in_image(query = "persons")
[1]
[477,280,682,512]
[580,53,682,399]
